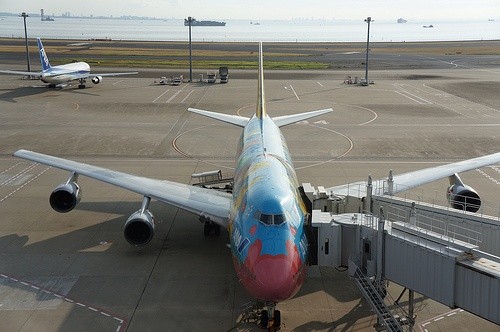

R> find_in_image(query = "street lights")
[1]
[19,11,31,71]
[184,16,196,80]
[364,18,374,80]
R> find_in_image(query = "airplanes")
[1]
[1,36,139,90]
[11,41,500,331]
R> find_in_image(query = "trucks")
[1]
[219,67,228,83]
[206,70,217,83]
